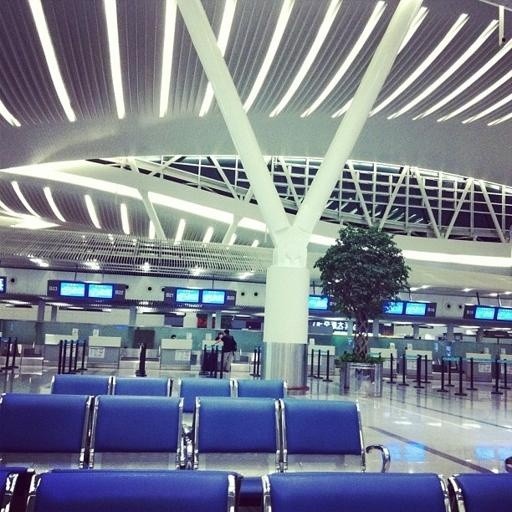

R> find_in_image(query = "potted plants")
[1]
[313,222,413,398]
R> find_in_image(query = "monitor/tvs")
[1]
[384,300,427,317]
[309,295,329,311]
[0,277,5,293]
[175,287,226,305]
[59,281,114,300]
[473,305,512,322]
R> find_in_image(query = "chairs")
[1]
[444,468,511,510]
[278,397,394,472]
[261,469,456,511]
[111,376,174,396]
[177,376,234,400]
[2,461,31,510]
[194,392,282,467]
[1,389,96,467]
[233,377,290,399]
[88,393,190,470]
[51,374,110,393]
[23,466,260,511]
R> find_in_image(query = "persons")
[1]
[171,335,177,338]
[215,329,236,372]
[215,332,224,341]
